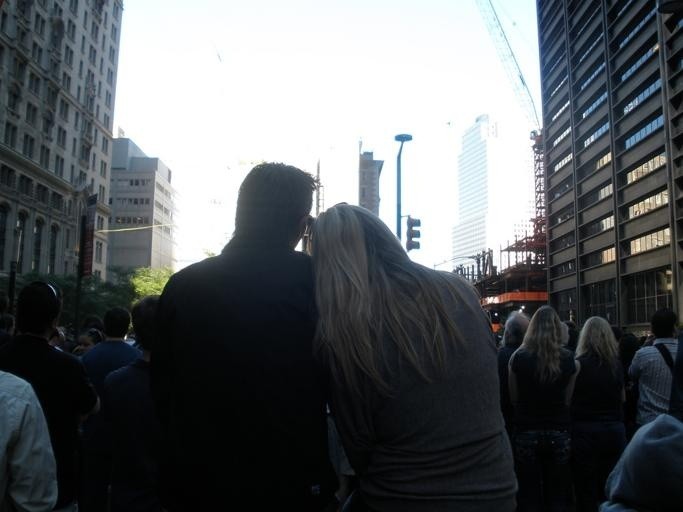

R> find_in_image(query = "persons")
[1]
[484,305,681,510]
[2,281,160,510]
[148,165,344,510]
[310,202,518,509]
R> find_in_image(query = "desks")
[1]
[390,134,414,241]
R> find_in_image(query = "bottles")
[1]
[17,281,59,329]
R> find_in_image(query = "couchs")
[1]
[403,213,421,254]
